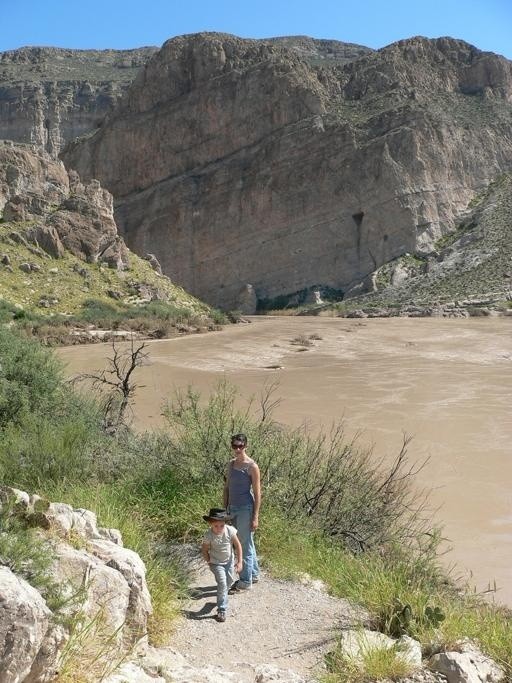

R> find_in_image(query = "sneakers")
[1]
[217,611,226,622]
[252,576,260,583]
[228,586,247,595]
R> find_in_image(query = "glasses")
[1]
[231,443,244,450]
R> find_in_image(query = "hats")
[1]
[202,508,234,522]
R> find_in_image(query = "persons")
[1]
[200,506,245,621]
[222,432,262,594]
